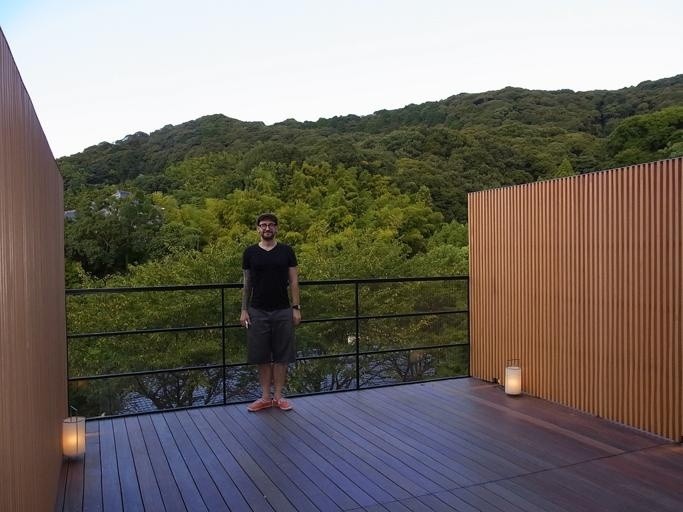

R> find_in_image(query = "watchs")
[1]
[291,304,301,310]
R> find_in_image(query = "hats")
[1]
[258,213,278,224]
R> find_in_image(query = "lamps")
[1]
[61,405,87,462]
[504,357,524,397]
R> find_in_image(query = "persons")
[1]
[237,213,302,413]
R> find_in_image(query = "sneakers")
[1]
[247,398,293,411]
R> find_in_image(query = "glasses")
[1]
[259,224,276,229]
